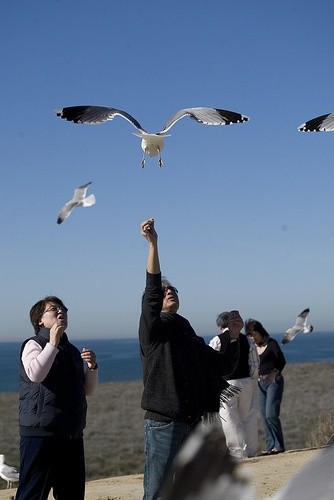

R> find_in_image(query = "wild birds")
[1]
[298,112,334,133]
[281,307,313,345]
[0,454,20,489]
[54,105,252,169]
[56,181,96,226]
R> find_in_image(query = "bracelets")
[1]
[87,363,98,370]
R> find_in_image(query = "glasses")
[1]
[162,284,179,293]
[42,304,67,314]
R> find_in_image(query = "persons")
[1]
[138,218,244,500]
[244,318,287,456]
[208,312,261,459]
[15,296,98,500]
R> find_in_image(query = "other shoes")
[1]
[269,446,284,455]
[258,447,270,457]
